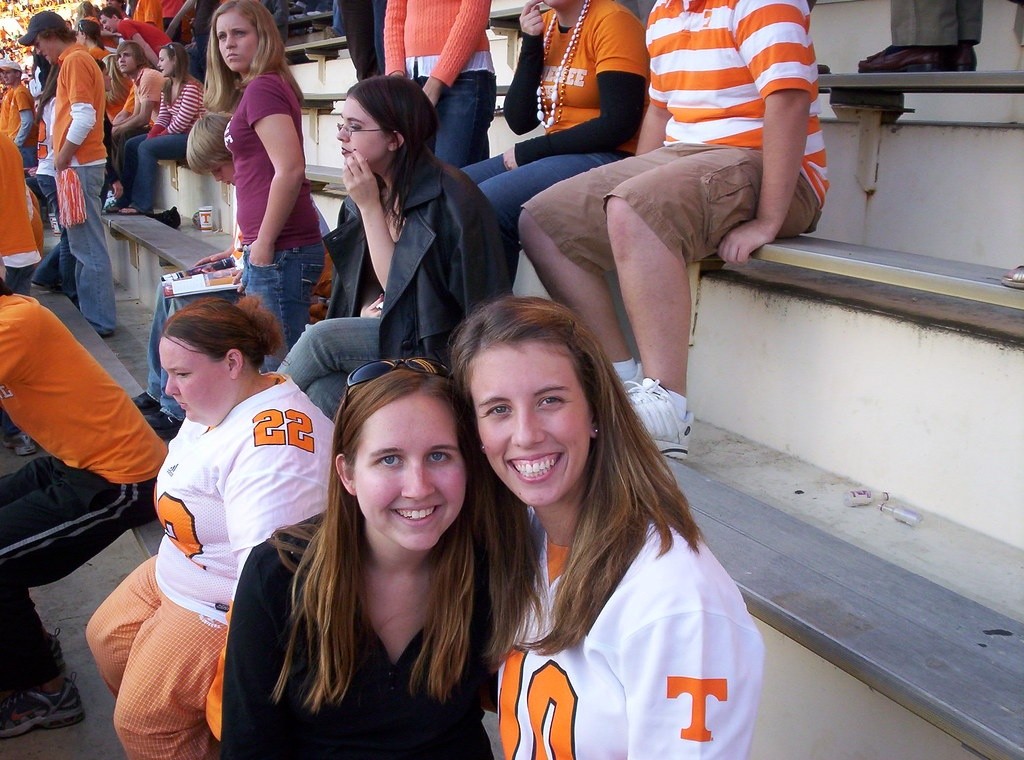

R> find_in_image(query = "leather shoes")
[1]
[858,45,946,71]
[946,42,976,71]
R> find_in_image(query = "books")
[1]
[160,257,243,298]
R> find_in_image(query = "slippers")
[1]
[106,206,121,212]
[117,207,141,215]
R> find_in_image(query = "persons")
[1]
[457,0,650,288]
[382,0,497,169]
[199,0,324,376]
[450,295,765,760]
[130,113,334,441]
[86,298,337,760]
[0,0,385,339]
[514,0,828,458]
[278,68,515,423]
[0,278,168,738]
[218,355,543,760]
[858,0,983,71]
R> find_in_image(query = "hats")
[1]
[18,11,66,46]
[0,62,22,72]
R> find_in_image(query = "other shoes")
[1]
[31,281,65,293]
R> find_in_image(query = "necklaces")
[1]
[535,0,590,130]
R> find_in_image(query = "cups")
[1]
[192,207,213,232]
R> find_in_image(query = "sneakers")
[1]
[145,411,183,437]
[2,432,36,455]
[102,197,117,210]
[131,392,162,414]
[0,627,65,697]
[624,377,695,461]
[0,672,85,738]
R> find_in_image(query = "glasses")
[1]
[337,123,400,137]
[344,358,453,411]
[78,20,89,39]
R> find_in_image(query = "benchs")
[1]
[20,0,1024,760]
